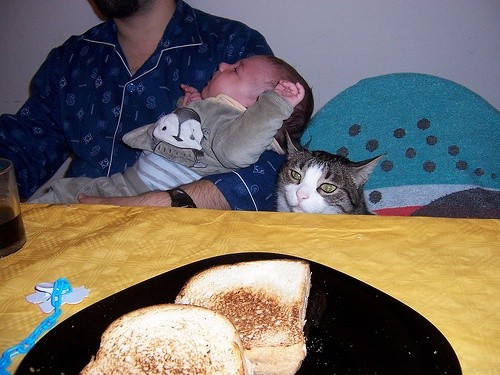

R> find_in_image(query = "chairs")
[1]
[300,73,500,219]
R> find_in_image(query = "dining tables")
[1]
[0,203,498,373]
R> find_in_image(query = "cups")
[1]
[0,158,27,257]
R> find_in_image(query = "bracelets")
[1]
[166,187,197,208]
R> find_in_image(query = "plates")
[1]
[10,252,466,375]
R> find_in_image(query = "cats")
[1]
[276,128,387,215]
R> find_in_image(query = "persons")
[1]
[0,0,285,211]
[22,54,314,202]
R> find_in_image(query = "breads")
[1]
[175,259,311,375]
[79,303,253,375]
